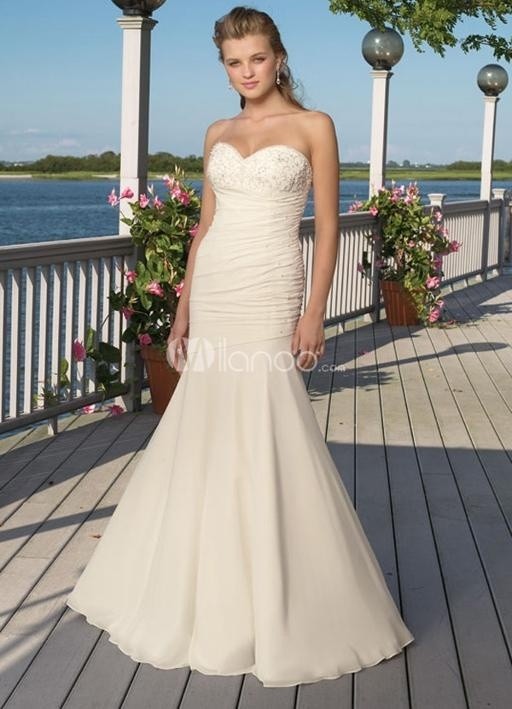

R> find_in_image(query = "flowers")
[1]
[348,179,462,329]
[32,165,202,417]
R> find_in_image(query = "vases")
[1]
[140,345,181,412]
[380,280,419,325]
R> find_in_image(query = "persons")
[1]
[66,4,415,689]
